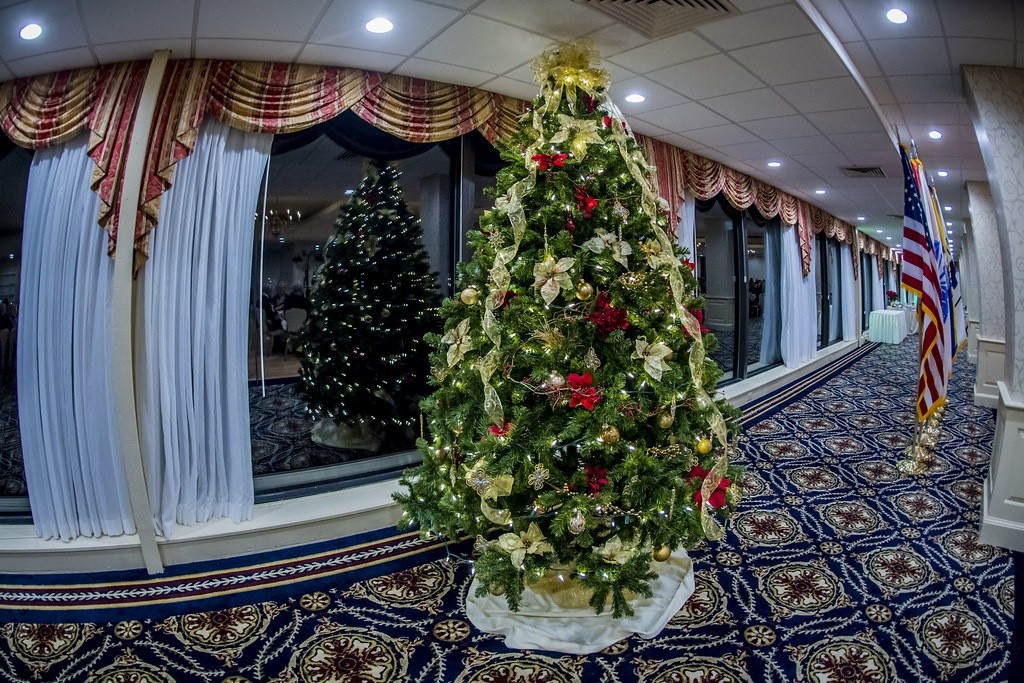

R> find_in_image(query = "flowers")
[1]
[886,290,898,301]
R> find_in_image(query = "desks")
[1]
[886,304,919,335]
[869,309,908,344]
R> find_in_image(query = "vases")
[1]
[889,298,895,305]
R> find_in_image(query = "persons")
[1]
[748,277,766,320]
[253,275,311,358]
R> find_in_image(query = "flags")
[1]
[899,132,969,421]
